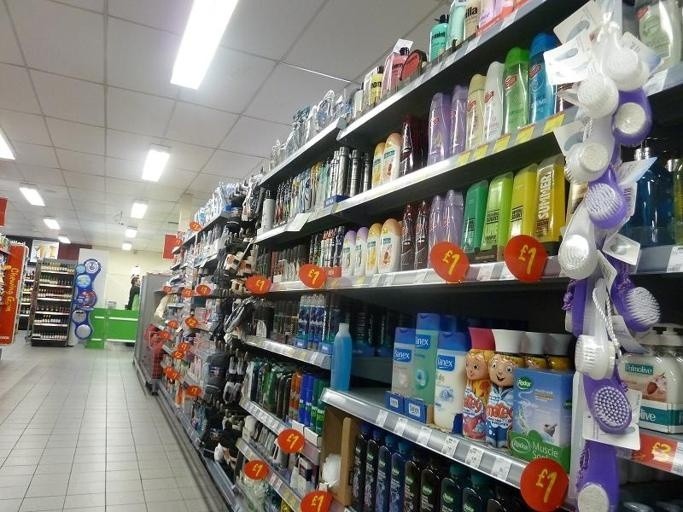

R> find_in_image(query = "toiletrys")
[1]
[342,0,522,113]
[343,2,682,202]
[341,136,682,275]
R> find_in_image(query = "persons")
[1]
[127,277,140,310]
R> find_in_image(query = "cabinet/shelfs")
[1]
[158,0,683,512]
[0,232,77,347]
